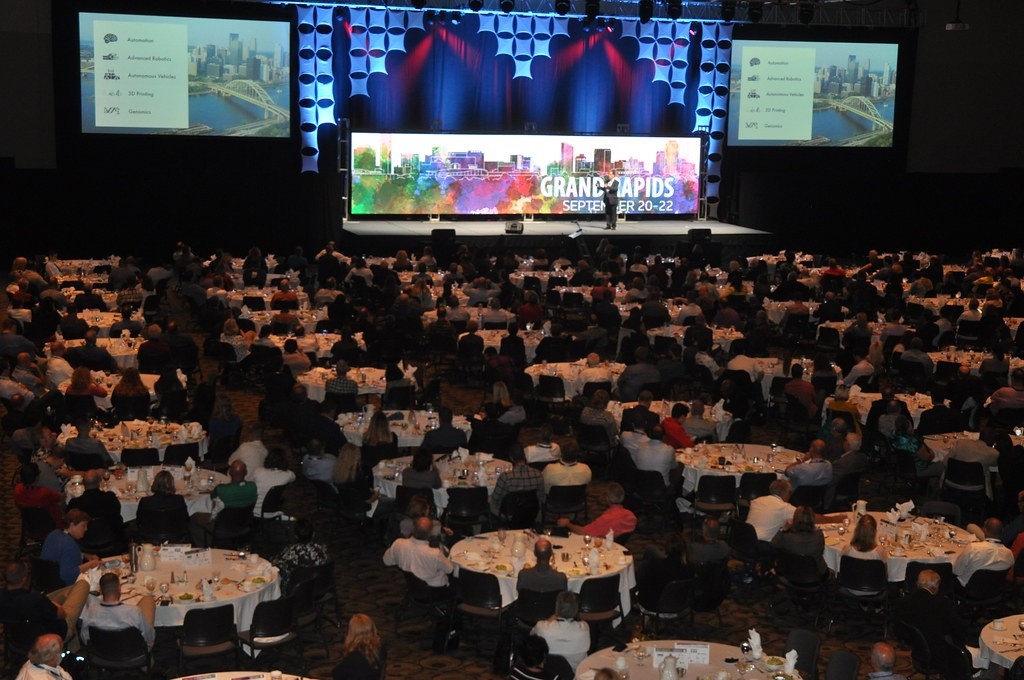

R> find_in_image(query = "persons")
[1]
[0,241,1024,680]
[595,170,619,230]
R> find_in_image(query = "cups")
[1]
[894,547,903,557]
[594,536,602,550]
[583,532,592,546]
[993,618,1005,628]
[617,656,626,668]
[250,553,259,565]
[562,552,573,563]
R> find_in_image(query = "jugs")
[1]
[658,653,680,680]
[136,543,157,571]
[851,500,868,523]
[510,532,529,558]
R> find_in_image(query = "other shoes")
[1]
[612,226,615,230]
[605,226,611,229]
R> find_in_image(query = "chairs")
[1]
[0,248,1024,680]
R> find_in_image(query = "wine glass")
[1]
[103,472,111,490]
[740,641,751,662]
[636,651,646,667]
[126,478,135,497]
[146,578,157,598]
[159,582,169,600]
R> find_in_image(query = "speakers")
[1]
[688,229,712,242]
[431,229,456,240]
[506,222,524,233]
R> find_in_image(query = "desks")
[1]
[753,358,844,401]
[63,260,116,274]
[1003,317,1024,341]
[44,338,149,370]
[816,322,917,350]
[693,268,729,285]
[336,410,473,455]
[525,362,626,400]
[171,671,311,680]
[449,530,636,628]
[77,312,146,338]
[927,352,1024,386]
[878,253,920,262]
[458,330,548,364]
[814,512,980,582]
[230,288,312,310]
[61,274,110,290]
[65,466,230,524]
[620,302,682,325]
[573,640,803,680]
[746,255,814,266]
[979,614,1024,669]
[66,290,120,311]
[942,265,967,283]
[675,443,812,497]
[296,366,419,404]
[59,373,160,409]
[716,281,777,302]
[491,257,535,271]
[58,421,209,465]
[255,333,366,362]
[423,308,517,330]
[870,281,912,299]
[822,393,952,429]
[646,256,675,266]
[762,301,850,324]
[230,273,289,290]
[647,326,744,354]
[908,298,984,315]
[509,271,575,290]
[240,310,329,333]
[75,547,281,658]
[365,257,420,271]
[613,401,733,443]
[398,271,445,288]
[203,259,278,273]
[798,268,860,288]
[372,454,513,518]
[554,287,629,304]
[922,432,1024,488]
[433,287,493,307]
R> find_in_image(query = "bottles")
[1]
[128,537,138,573]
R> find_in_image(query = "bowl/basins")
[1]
[171,592,199,604]
[763,656,786,671]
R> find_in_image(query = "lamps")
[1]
[582,17,617,32]
[428,10,462,25]
[690,22,699,35]
[469,0,814,24]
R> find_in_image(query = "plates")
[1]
[990,623,1008,630]
[491,562,513,574]
[720,656,740,666]
